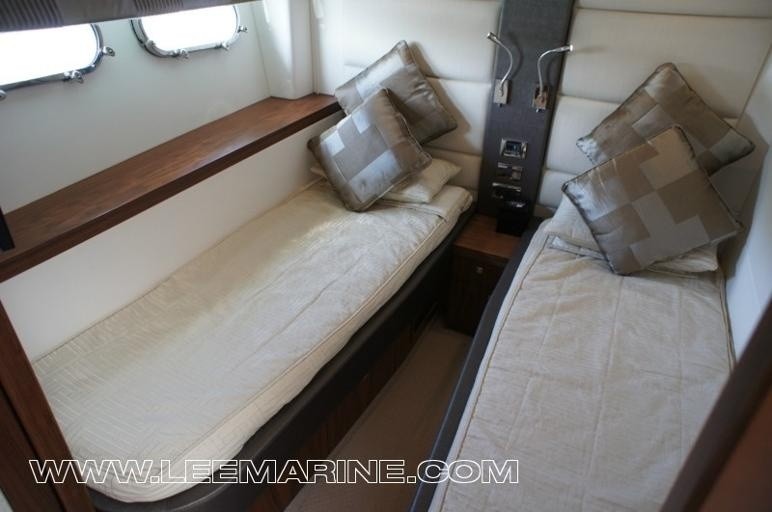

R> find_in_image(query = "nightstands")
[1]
[446,215,525,338]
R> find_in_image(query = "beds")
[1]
[0,92,472,511]
[410,210,737,512]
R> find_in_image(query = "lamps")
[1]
[530,43,574,112]
[486,31,514,106]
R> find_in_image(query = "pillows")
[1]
[577,63,755,177]
[546,193,718,274]
[311,158,460,205]
[307,90,430,212]
[560,126,746,275]
[333,40,457,145]
